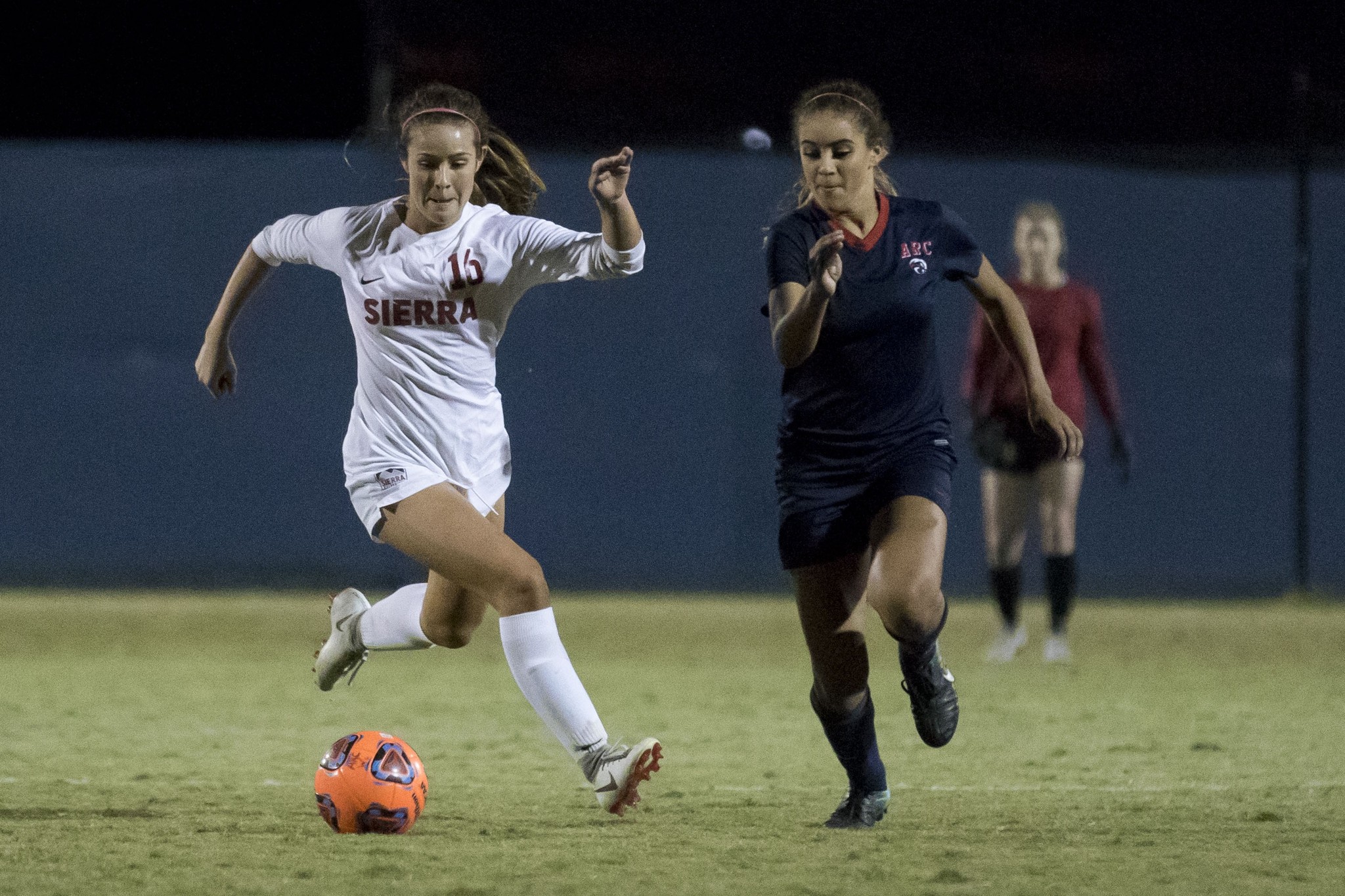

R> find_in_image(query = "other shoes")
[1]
[984,621,1028,661]
[1041,633,1070,664]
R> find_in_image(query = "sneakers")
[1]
[576,737,664,817]
[311,586,373,692]
[821,760,891,830]
[899,640,959,748]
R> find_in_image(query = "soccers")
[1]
[311,729,430,836]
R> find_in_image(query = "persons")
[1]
[960,196,1143,667]
[760,81,1086,830]
[190,85,670,820]
